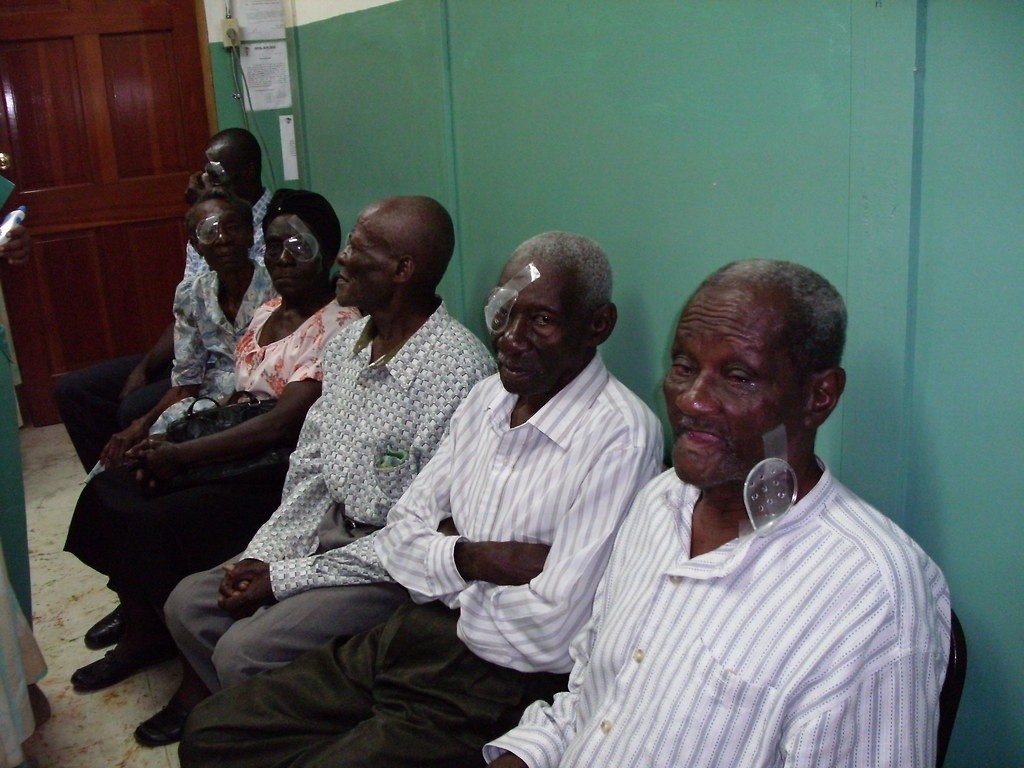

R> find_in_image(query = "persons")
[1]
[0,209,52,727]
[164,196,496,695]
[62,127,275,651]
[63,189,363,747]
[483,258,952,768]
[177,233,662,768]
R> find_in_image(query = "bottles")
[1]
[0,205,27,246]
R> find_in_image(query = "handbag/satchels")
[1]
[167,391,290,484]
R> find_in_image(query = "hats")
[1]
[261,188,342,271]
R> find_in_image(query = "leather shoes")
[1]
[84,605,125,643]
[72,649,135,690]
[135,702,187,745]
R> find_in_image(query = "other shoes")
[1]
[27,683,51,724]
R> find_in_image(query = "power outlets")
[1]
[221,18,241,48]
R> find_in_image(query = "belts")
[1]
[343,511,370,530]
